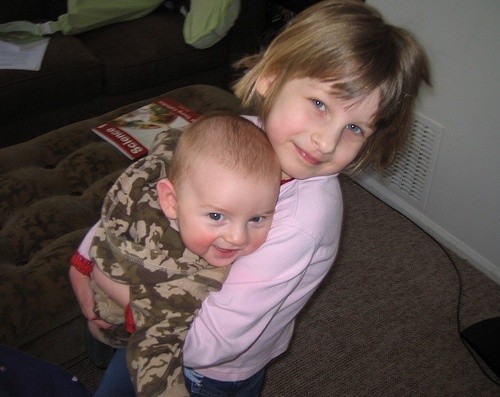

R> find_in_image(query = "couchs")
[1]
[0,0,265,148]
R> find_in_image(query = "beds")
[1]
[0,1,500,397]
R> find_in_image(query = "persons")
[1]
[88,113,281,397]
[69,0,434,397]
